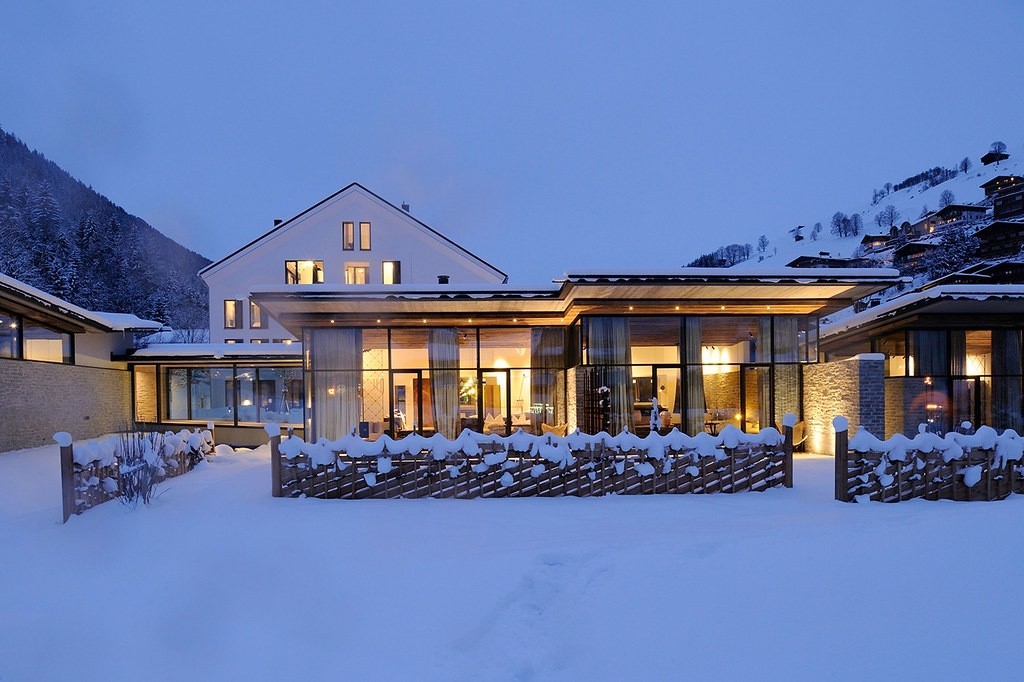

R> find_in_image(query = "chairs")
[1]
[511,414,531,431]
[483,413,505,436]
[542,423,569,437]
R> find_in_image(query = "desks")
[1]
[705,421,720,436]
